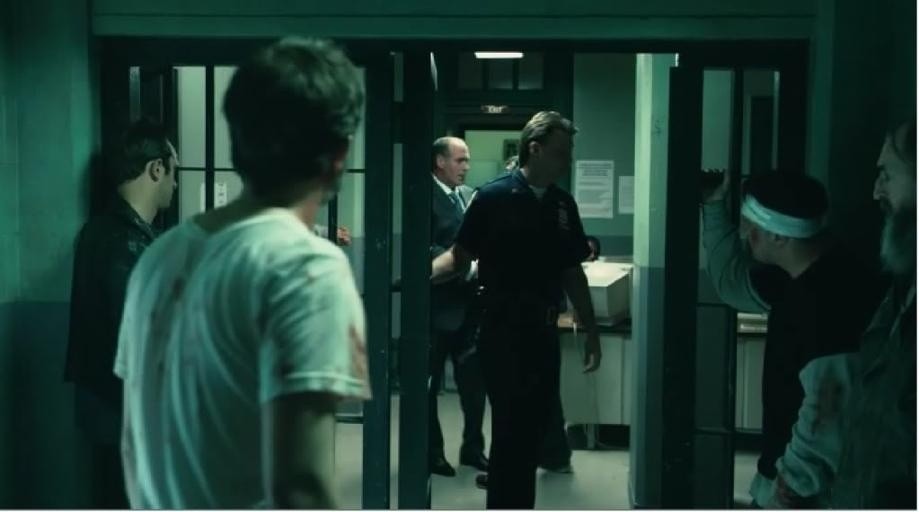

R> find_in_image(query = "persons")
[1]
[106,31,377,509]
[471,152,580,491]
[696,165,858,509]
[859,114,916,352]
[52,127,184,509]
[760,201,917,510]
[312,219,357,250]
[389,109,606,508]
[413,133,492,478]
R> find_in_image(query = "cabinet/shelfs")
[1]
[560,333,766,426]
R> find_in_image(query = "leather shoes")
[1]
[459,443,492,472]
[430,455,456,477]
